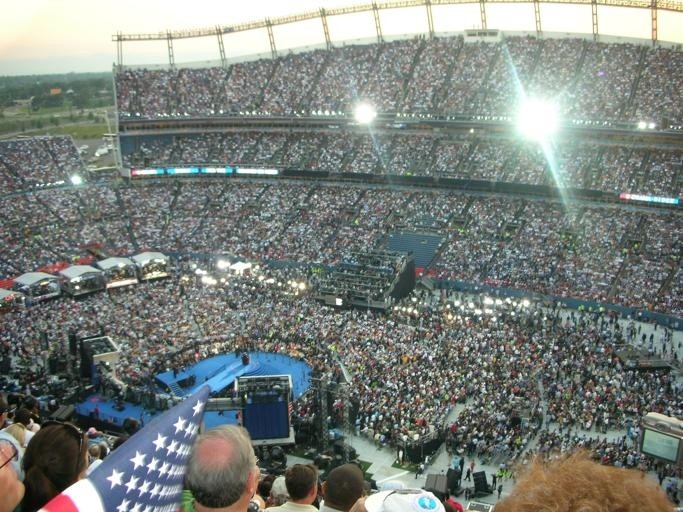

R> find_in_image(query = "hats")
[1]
[364,488,445,512]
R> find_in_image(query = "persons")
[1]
[2,32,683,512]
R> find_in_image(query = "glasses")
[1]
[40,420,84,454]
[0,438,19,469]
